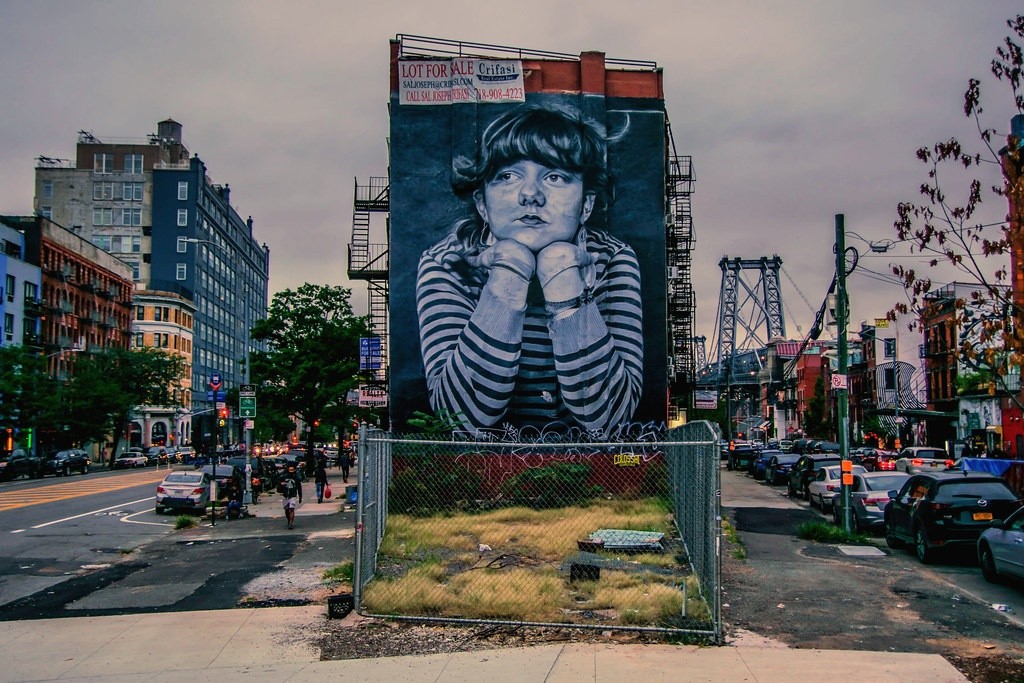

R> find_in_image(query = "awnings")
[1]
[867,408,959,418]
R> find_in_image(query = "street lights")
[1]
[178,238,253,504]
[848,328,902,441]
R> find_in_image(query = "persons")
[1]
[961,443,1009,459]
[339,451,349,483]
[350,450,355,468]
[280,465,302,529]
[101,447,107,466]
[315,465,328,504]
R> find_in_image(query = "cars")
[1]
[0,449,36,483]
[831,471,917,535]
[719,436,900,515]
[977,505,1024,583]
[130,439,359,491]
[197,464,247,502]
[895,447,955,476]
[155,470,222,516]
[113,452,148,469]
[44,448,91,477]
[884,470,1024,565]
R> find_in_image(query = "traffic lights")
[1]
[222,409,226,419]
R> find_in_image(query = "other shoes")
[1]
[318,499,323,503]
[288,524,293,530]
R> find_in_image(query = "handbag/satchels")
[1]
[277,474,286,493]
[325,486,331,499]
